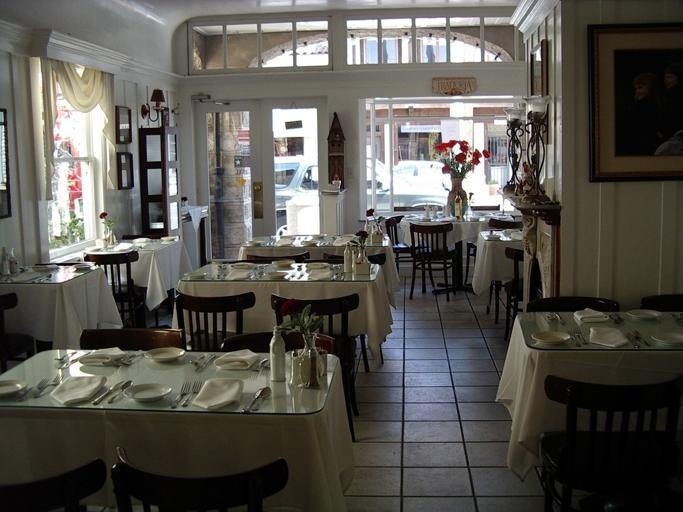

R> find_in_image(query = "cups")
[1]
[96,239,108,248]
[318,346,327,376]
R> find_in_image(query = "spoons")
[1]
[108,380,134,402]
[246,387,272,412]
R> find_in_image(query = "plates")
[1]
[306,263,328,269]
[272,261,295,266]
[486,234,501,239]
[161,237,176,240]
[529,331,570,344]
[268,272,288,278]
[123,382,172,401]
[184,272,208,278]
[627,310,662,318]
[74,264,91,269]
[79,356,111,363]
[650,332,683,346]
[144,347,185,361]
[511,232,523,241]
[231,264,253,269]
[0,379,27,394]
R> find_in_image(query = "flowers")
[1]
[433,140,491,177]
[99,212,115,230]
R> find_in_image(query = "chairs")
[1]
[528,296,621,312]
[541,376,683,510]
[112,445,288,512]
[272,292,360,417]
[0,459,107,512]
[643,294,682,312]
[385,215,525,341]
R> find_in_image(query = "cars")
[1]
[274,147,501,232]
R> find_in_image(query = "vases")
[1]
[106,228,114,245]
[279,304,324,389]
[448,177,469,217]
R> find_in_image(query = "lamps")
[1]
[141,89,166,121]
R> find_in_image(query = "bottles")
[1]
[2,247,10,275]
[344,242,352,272]
[455,194,462,219]
[269,327,285,381]
[301,334,319,388]
[10,248,17,273]
[289,344,302,388]
[332,174,342,189]
[356,248,369,263]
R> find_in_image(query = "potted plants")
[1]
[48,209,85,250]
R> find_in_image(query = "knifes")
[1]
[92,380,124,406]
[57,352,92,368]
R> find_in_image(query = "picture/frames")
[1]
[116,105,132,144]
[529,40,547,97]
[588,23,682,182]
[117,151,133,188]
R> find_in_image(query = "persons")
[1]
[616,64,683,155]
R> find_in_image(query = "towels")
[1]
[191,377,243,410]
[587,327,629,348]
[51,375,108,404]
[573,307,609,322]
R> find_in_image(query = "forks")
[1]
[574,327,587,344]
[182,380,202,405]
[32,374,64,398]
[171,381,191,408]
[15,376,49,401]
